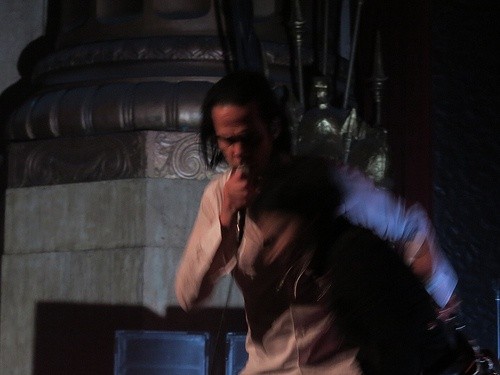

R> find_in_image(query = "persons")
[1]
[244,154,479,374]
[172,72,457,375]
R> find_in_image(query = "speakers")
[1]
[226,334,248,375]
[114,330,211,375]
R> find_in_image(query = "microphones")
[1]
[235,209,247,245]
